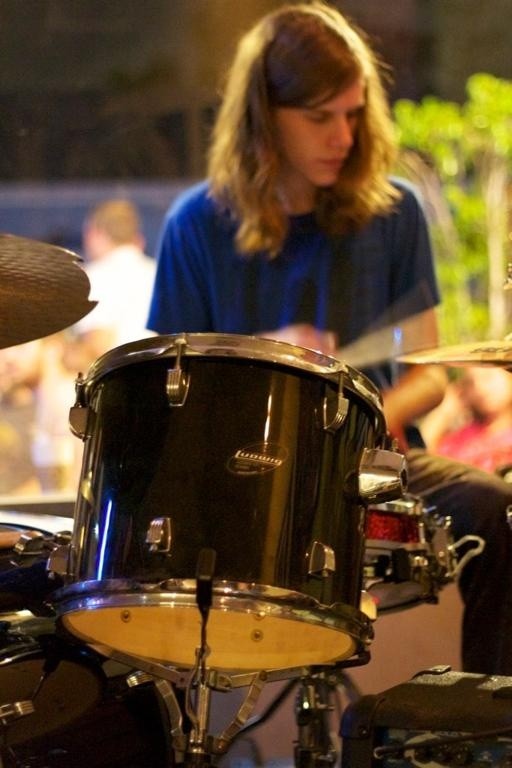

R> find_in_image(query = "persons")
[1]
[141,2,511,764]
[31,199,158,506]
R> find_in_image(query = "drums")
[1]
[56,330,374,682]
[361,496,443,612]
[0,525,104,748]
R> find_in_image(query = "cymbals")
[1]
[0,232,98,355]
[394,339,512,370]
